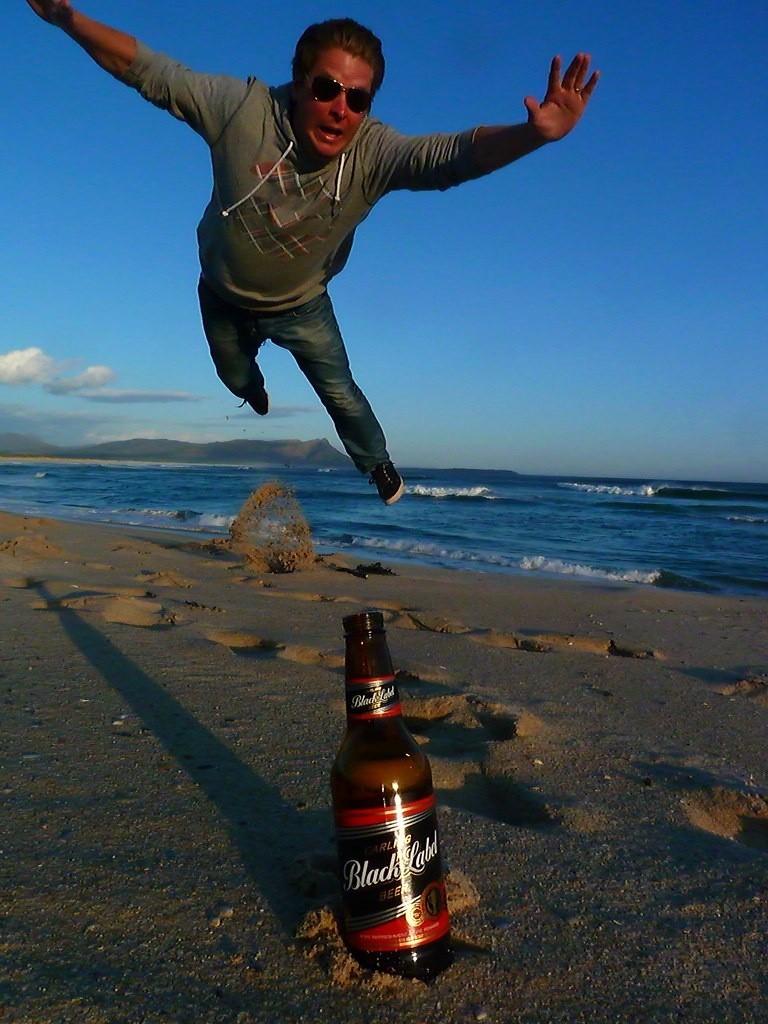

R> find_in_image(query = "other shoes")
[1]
[244,384,270,416]
[368,462,404,506]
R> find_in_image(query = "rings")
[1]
[576,88,579,91]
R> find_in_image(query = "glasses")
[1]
[305,77,371,115]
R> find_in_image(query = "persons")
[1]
[25,0,600,503]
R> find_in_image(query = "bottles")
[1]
[330,611,457,981]
